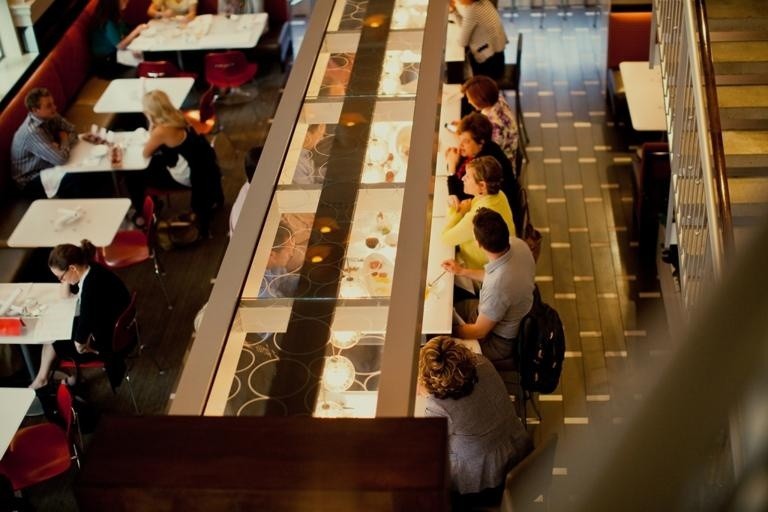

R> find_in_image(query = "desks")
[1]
[56,131,153,198]
[0,387,37,461]
[303,33,463,417]
[94,195,172,310]
[6,198,132,246]
[94,77,196,113]
[2,282,79,417]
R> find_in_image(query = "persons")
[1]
[85,0,268,80]
[453,208,536,371]
[142,89,197,194]
[229,77,345,340]
[445,112,523,238]
[10,87,79,204]
[450,0,509,79]
[418,336,537,512]
[451,75,518,174]
[440,155,515,302]
[27,239,131,390]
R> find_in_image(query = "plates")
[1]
[141,25,157,37]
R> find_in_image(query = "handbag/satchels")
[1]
[36,385,97,432]
[170,224,200,246]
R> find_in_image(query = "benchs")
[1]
[123,0,294,72]
[606,13,654,148]
[630,143,669,235]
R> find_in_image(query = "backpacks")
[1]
[514,303,565,395]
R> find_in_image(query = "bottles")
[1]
[108,143,124,168]
[138,78,147,103]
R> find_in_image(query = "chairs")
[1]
[186,86,223,148]
[502,431,558,510]
[59,290,147,418]
[3,384,84,499]
[496,33,531,164]
[490,315,542,431]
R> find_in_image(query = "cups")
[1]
[365,232,380,252]
[223,8,233,21]
[384,170,395,182]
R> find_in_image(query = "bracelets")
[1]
[447,173,453,175]
